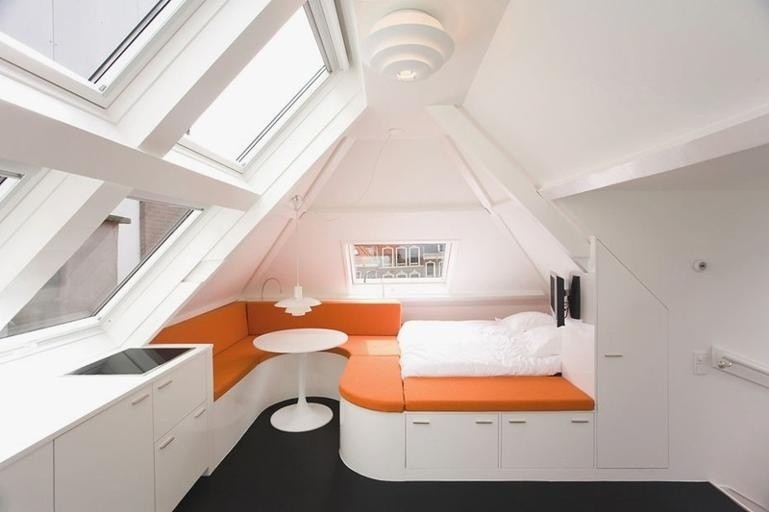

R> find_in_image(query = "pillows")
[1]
[495,312,563,358]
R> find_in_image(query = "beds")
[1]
[400,310,577,377]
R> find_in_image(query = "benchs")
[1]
[149,300,247,479]
[248,301,401,428]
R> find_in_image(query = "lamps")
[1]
[273,195,322,317]
[360,13,453,86]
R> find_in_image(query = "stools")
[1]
[404,375,595,483]
[339,355,406,482]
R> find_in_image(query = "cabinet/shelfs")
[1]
[0,352,209,510]
[406,412,594,470]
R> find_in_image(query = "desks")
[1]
[252,326,348,435]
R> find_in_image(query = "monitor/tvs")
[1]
[549,270,565,328]
